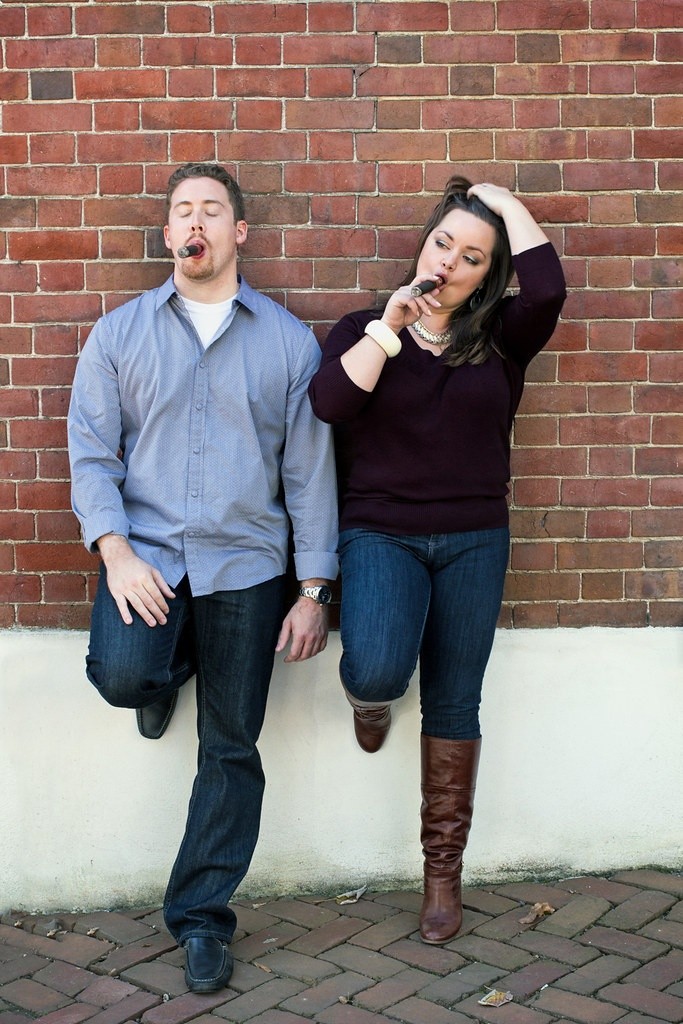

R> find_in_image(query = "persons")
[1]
[306,176,567,945]
[66,165,339,994]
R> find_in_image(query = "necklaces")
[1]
[412,321,452,345]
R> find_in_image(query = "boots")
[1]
[337,653,408,755]
[416,733,483,944]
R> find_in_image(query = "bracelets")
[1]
[364,320,402,357]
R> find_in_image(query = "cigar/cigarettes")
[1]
[178,245,201,257]
[411,276,442,297]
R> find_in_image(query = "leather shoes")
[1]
[134,686,179,740]
[183,936,234,994]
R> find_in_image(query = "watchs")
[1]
[299,586,332,606]
